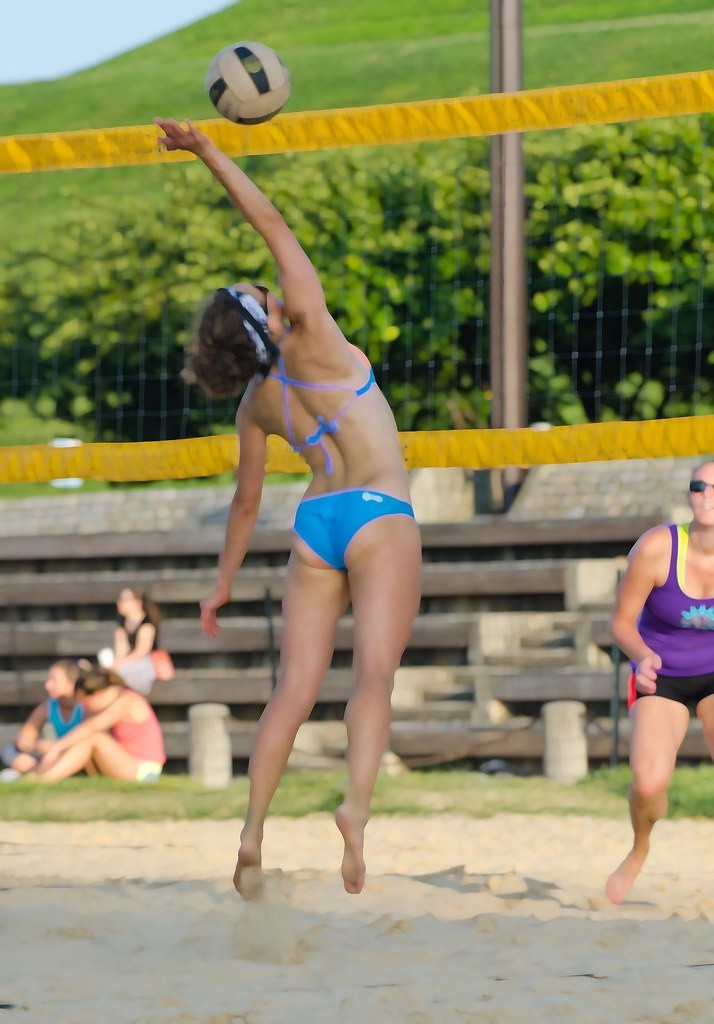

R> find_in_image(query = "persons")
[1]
[154,116,426,900]
[604,460,714,904]
[0,585,167,786]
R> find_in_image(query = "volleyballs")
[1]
[204,39,290,125]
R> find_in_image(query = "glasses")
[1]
[256,285,269,314]
[690,481,713,492]
